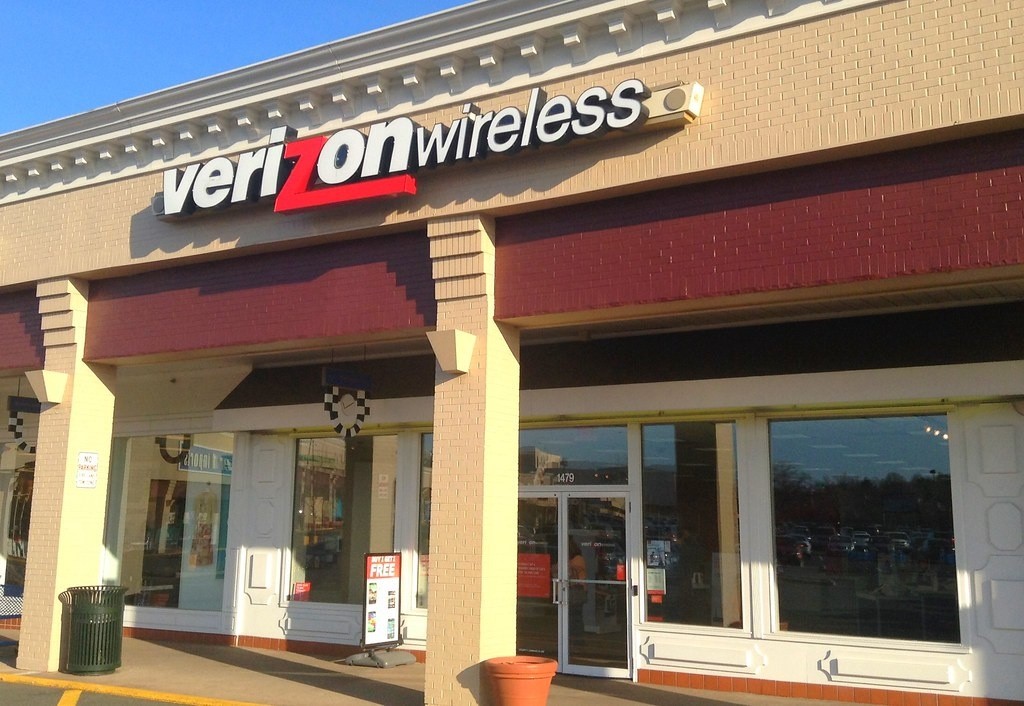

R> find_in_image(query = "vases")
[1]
[487,656,559,706]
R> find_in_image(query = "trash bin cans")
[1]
[67,585,130,676]
